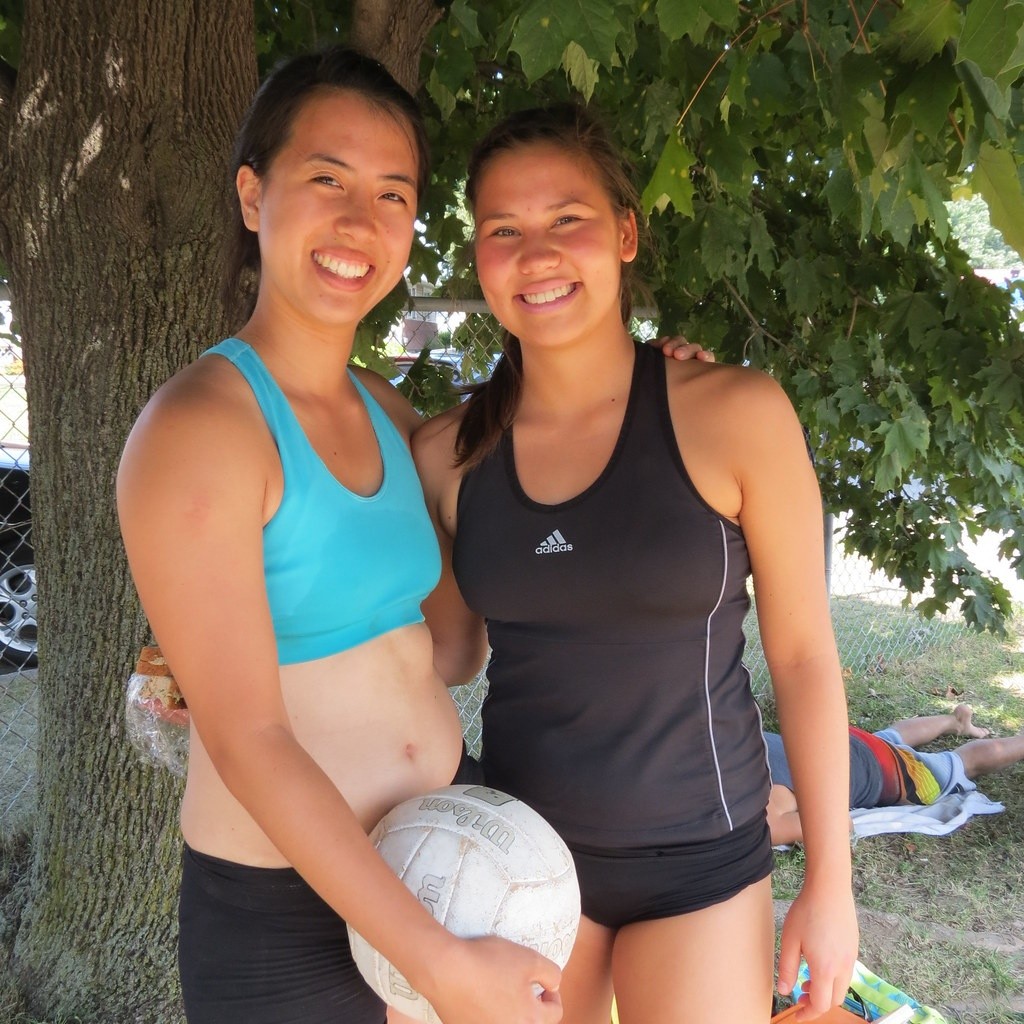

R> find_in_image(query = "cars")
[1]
[374,351,497,412]
[0,461,38,665]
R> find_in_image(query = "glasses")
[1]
[847,986,873,1022]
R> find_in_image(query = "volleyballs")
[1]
[345,783,582,1024]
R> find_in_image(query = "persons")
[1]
[115,38,715,1024]
[131,100,861,1023]
[763,704,1024,845]
